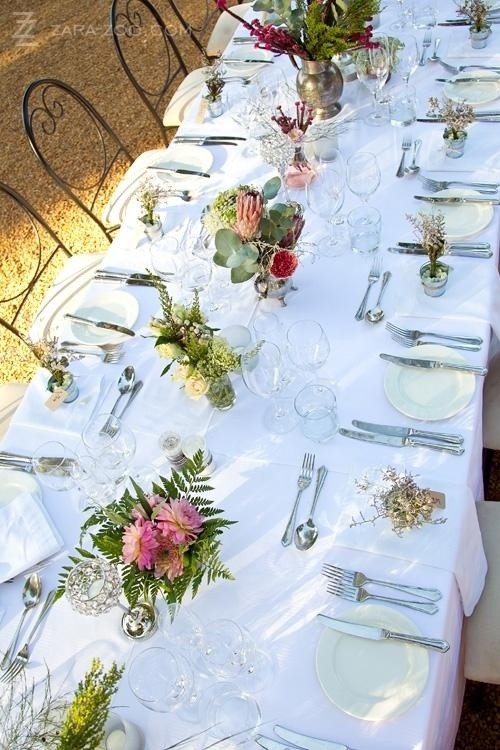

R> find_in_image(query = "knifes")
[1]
[387,247,493,259]
[445,17,500,22]
[414,195,500,205]
[380,353,489,377]
[96,269,170,282]
[254,734,300,750]
[0,451,80,467]
[416,118,499,123]
[223,59,275,64]
[338,427,465,456]
[316,614,450,653]
[95,275,167,289]
[174,140,238,146]
[147,166,211,178]
[66,314,136,337]
[273,725,352,749]
[0,461,73,477]
[396,242,490,249]
[175,135,246,141]
[438,21,500,26]
[352,420,464,444]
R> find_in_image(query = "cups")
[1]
[241,341,302,433]
[286,319,336,396]
[65,558,161,641]
[150,237,189,287]
[226,85,259,159]
[347,206,382,255]
[389,0,409,32]
[260,132,304,216]
[256,65,287,116]
[355,48,390,127]
[196,618,273,694]
[170,237,231,322]
[127,647,216,724]
[69,455,118,504]
[319,149,344,187]
[218,323,259,375]
[412,7,438,31]
[94,712,145,750]
[390,35,420,87]
[32,441,108,514]
[346,152,381,206]
[386,83,417,128]
[294,384,341,442]
[157,602,227,674]
[209,256,242,292]
[304,168,349,257]
[253,312,282,356]
[82,414,161,497]
[310,121,338,164]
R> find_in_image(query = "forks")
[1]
[59,349,127,364]
[417,174,498,189]
[419,31,432,66]
[220,72,256,80]
[396,135,412,177]
[0,589,57,685]
[281,452,315,547]
[354,255,383,321]
[102,380,144,440]
[320,563,443,602]
[385,321,483,345]
[390,332,481,352]
[439,60,500,70]
[422,183,498,195]
[61,341,126,353]
[325,581,439,615]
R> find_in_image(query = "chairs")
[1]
[0,183,104,359]
[112,2,214,146]
[171,0,254,67]
[22,81,168,245]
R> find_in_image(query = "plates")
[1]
[71,290,139,346]
[384,345,476,421]
[453,0,500,12]
[0,469,43,512]
[224,49,274,72]
[422,188,494,239]
[157,146,214,182]
[443,70,500,105]
[316,604,429,722]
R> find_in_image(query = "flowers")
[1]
[29,337,69,386]
[202,178,304,284]
[56,658,126,750]
[348,465,447,538]
[146,268,265,407]
[452,1,493,31]
[425,97,474,140]
[52,445,235,625]
[271,102,316,172]
[404,203,451,277]
[138,178,161,226]
[214,0,386,60]
[202,49,225,103]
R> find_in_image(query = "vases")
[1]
[208,101,223,118]
[420,261,449,297]
[204,373,236,411]
[469,25,489,49]
[445,130,465,158]
[392,513,415,530]
[254,271,293,300]
[143,220,164,242]
[48,372,79,404]
[296,57,343,120]
[284,146,319,188]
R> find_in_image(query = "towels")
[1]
[9,365,114,439]
[0,489,64,586]
[426,124,500,173]
[111,209,191,254]
[394,257,500,342]
[329,464,490,619]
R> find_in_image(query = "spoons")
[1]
[294,465,328,551]
[366,271,392,322]
[404,138,422,175]
[427,37,441,63]
[98,365,135,437]
[1,572,42,671]
[224,80,252,85]
